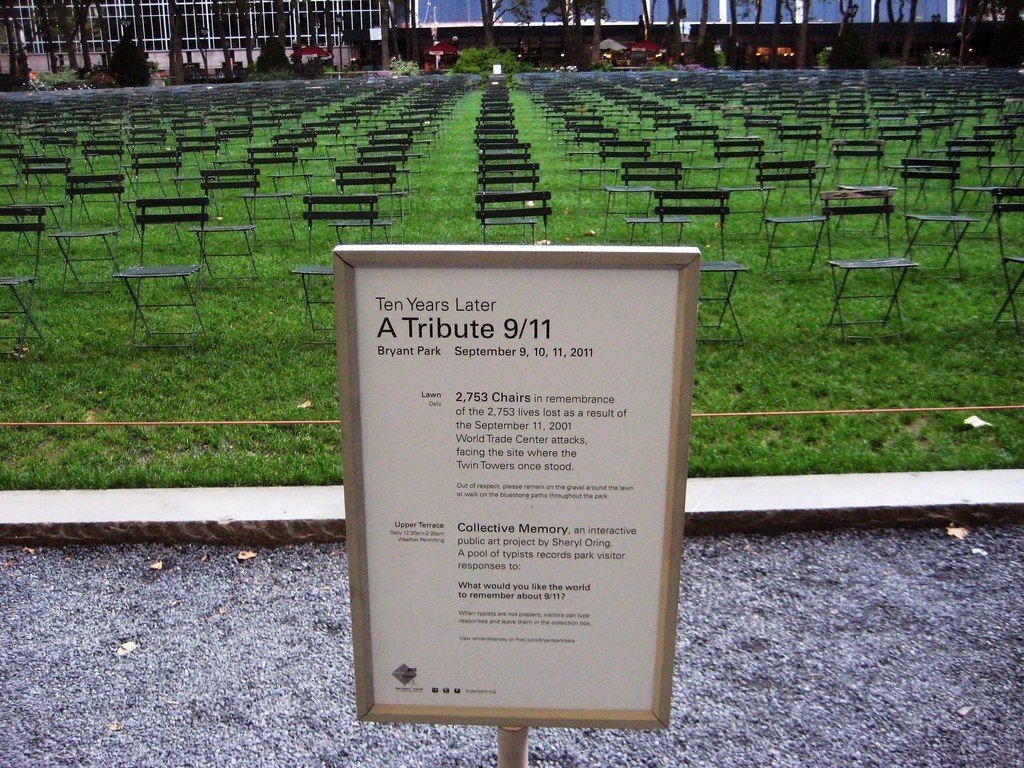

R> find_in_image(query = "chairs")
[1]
[0,66,1024,360]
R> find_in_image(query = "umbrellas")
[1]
[590,38,628,51]
[292,44,329,57]
[628,39,662,52]
[425,41,461,54]
